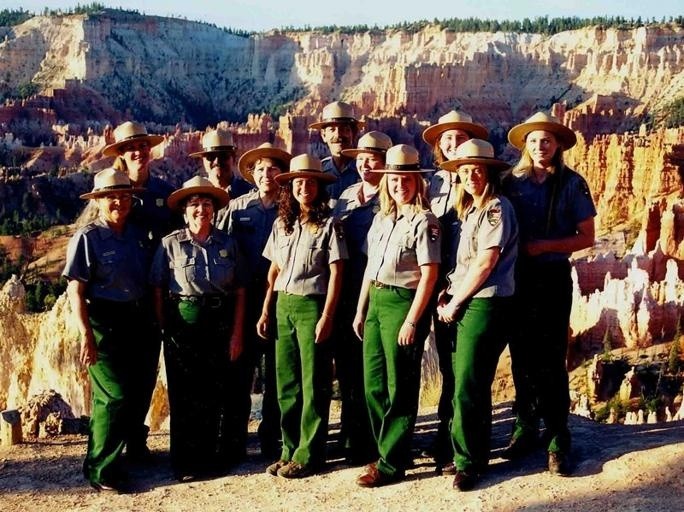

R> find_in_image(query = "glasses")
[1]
[456,167,485,175]
[206,152,229,161]
[106,192,130,201]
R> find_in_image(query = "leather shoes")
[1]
[90,481,119,492]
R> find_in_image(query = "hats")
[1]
[370,144,435,173]
[274,154,337,186]
[508,112,576,150]
[309,101,368,129]
[238,142,294,185]
[440,138,512,172]
[341,131,393,157]
[422,110,488,146]
[189,130,243,158]
[80,167,144,200]
[167,175,230,211]
[103,121,164,156]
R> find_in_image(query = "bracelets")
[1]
[450,299,460,309]
[322,312,333,320]
[403,319,417,329]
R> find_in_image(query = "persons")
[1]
[350,144,443,489]
[150,176,246,485]
[256,152,351,480]
[64,168,158,493]
[76,100,510,463]
[500,111,596,479]
[433,138,520,495]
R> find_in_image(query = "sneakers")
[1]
[276,461,310,479]
[364,463,376,472]
[266,459,288,477]
[501,439,519,460]
[356,464,388,486]
[435,461,456,476]
[453,471,484,492]
[548,450,568,476]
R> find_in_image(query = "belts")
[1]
[371,280,395,289]
[172,296,222,307]
